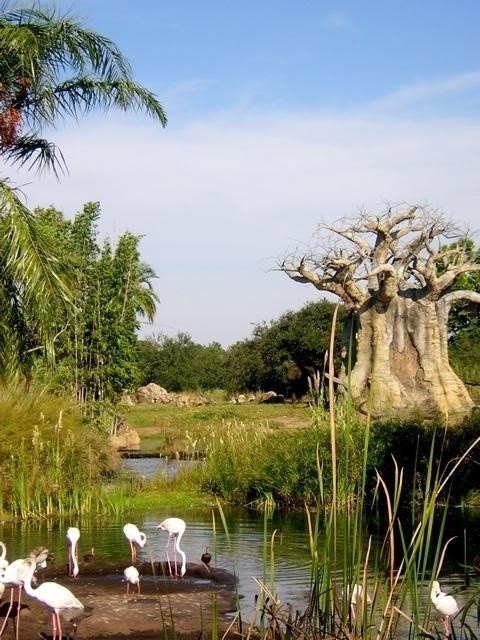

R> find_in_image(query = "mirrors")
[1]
[429,580,458,640]
[123,523,147,563]
[155,518,186,578]
[123,565,140,595]
[65,526,81,580]
[339,584,372,620]
[201,547,211,567]
[0,541,85,640]
[83,544,96,562]
